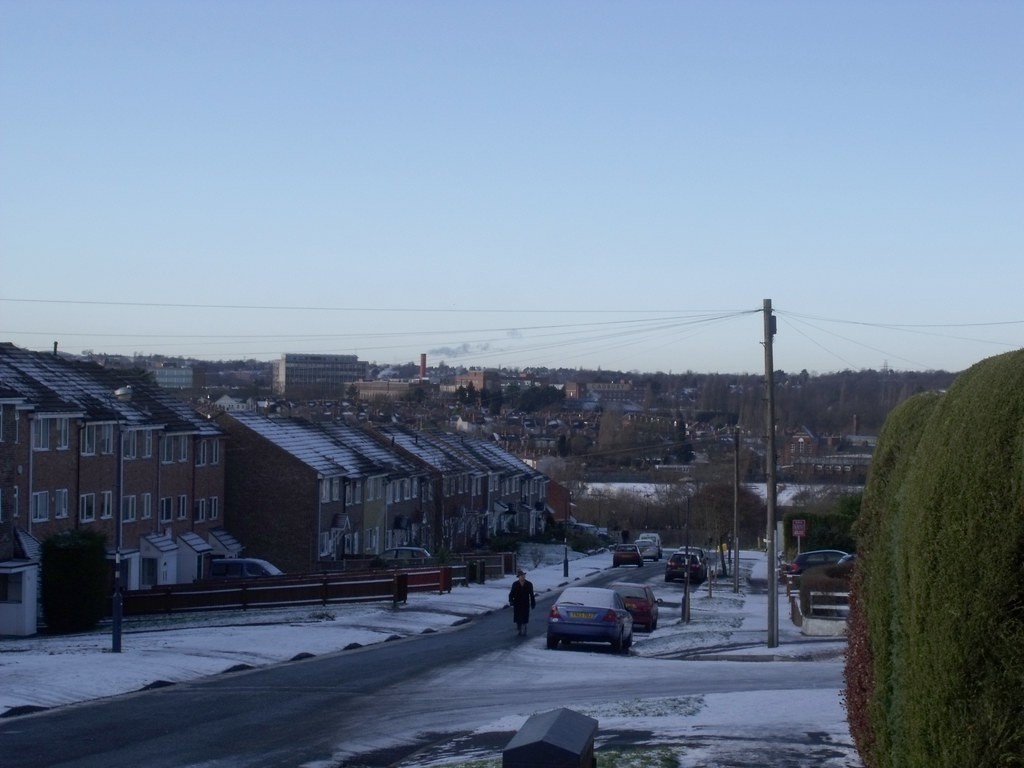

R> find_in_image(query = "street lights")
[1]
[563,490,574,576]
[105,387,134,654]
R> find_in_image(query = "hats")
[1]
[516,571,526,577]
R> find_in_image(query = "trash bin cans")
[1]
[500,705,598,767]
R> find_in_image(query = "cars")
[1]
[664,551,706,584]
[369,546,436,570]
[679,547,709,578]
[612,543,643,568]
[635,540,659,562]
[834,553,860,581]
[784,550,849,587]
[547,587,635,652]
[609,582,664,633]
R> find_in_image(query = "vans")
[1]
[639,532,663,558]
[208,557,284,582]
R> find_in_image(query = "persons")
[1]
[621,527,630,544]
[509,571,536,635]
